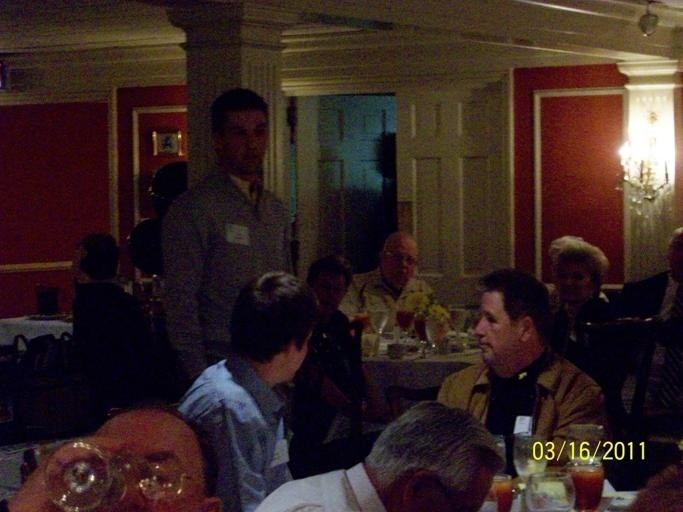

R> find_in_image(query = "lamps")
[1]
[617,140,673,202]
[638,0,662,37]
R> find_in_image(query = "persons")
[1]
[363,131,397,274]
[122,159,188,281]
[2,229,683,512]
[156,87,300,405]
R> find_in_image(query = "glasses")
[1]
[383,249,417,264]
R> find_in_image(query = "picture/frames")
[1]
[151,130,183,156]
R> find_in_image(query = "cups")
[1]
[365,305,391,333]
[513,431,549,476]
[566,457,606,512]
[42,441,127,512]
[480,473,514,512]
[524,469,577,512]
